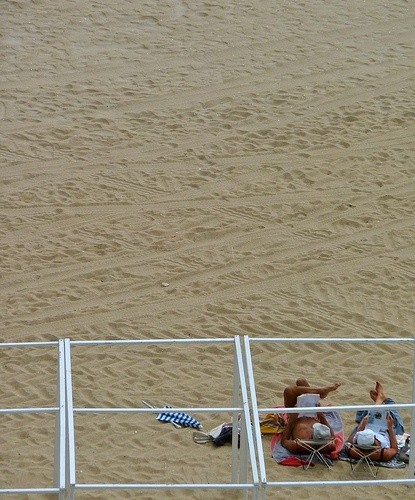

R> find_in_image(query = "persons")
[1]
[345,381,397,461]
[280,377,342,455]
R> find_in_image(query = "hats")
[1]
[356,429,375,446]
[313,423,331,441]
